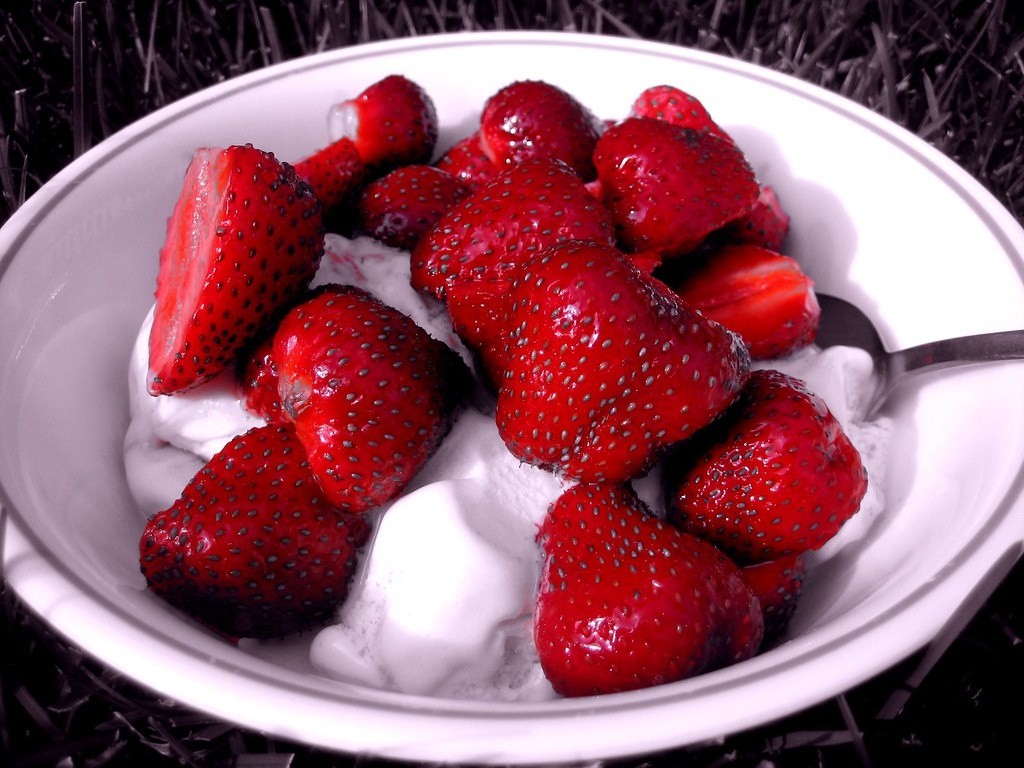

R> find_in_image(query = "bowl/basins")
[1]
[0,29,1024,768]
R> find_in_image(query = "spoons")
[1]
[805,290,1024,423]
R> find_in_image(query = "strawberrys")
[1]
[130,71,868,687]
